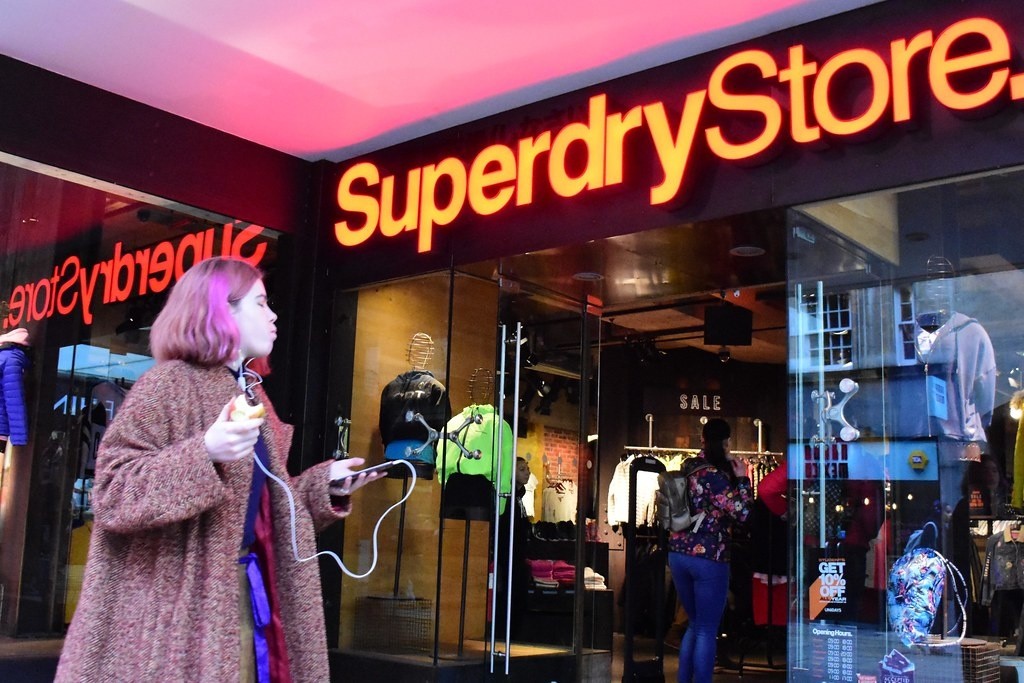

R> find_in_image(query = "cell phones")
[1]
[328,460,395,489]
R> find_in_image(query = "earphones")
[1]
[237,376,246,392]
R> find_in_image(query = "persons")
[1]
[494,456,536,643]
[912,499,953,558]
[432,367,515,525]
[951,453,1010,643]
[378,330,454,480]
[667,416,754,683]
[50,258,387,683]
[756,436,888,629]
[894,246,1004,487]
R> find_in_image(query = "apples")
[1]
[226,395,266,421]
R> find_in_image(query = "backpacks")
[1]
[656,462,716,532]
